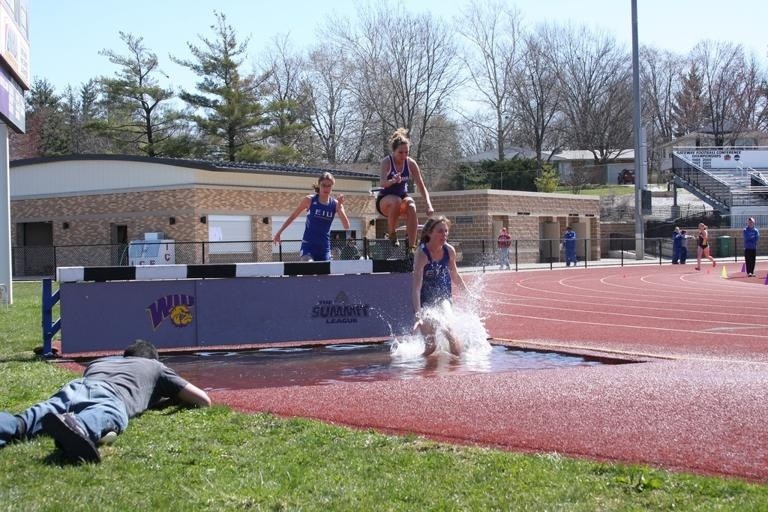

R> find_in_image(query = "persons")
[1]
[409,214,472,358]
[742,217,760,278]
[561,227,577,268]
[671,226,686,264]
[341,237,361,260]
[272,171,350,274]
[680,229,695,264]
[694,222,717,271]
[417,357,462,376]
[497,227,512,270]
[0,336,212,465]
[375,127,434,256]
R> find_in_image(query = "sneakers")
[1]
[42,412,101,465]
[406,245,417,265]
[388,232,399,247]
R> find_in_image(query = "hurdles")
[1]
[40,257,424,361]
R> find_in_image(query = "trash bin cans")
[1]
[717,236,732,257]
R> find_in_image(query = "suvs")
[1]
[618,168,635,184]
